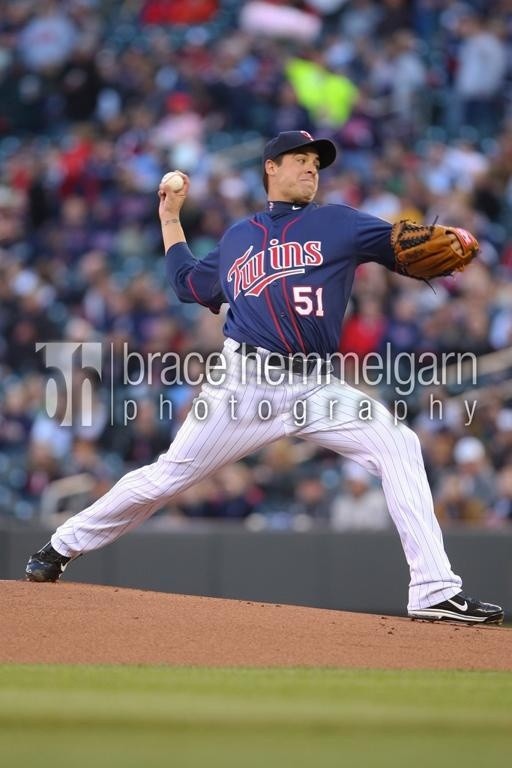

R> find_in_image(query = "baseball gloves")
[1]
[389,217,478,279]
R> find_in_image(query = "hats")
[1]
[262,130,336,171]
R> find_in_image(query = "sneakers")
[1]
[25,539,72,582]
[407,590,504,625]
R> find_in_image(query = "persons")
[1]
[23,129,507,625]
[0,0,512,529]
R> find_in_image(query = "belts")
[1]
[236,345,326,375]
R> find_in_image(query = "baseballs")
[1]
[161,171,184,193]
